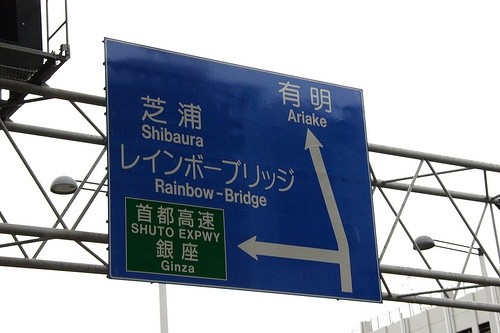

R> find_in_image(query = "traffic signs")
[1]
[101,36,383,304]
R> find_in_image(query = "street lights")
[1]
[413,234,495,333]
[50,176,169,333]
